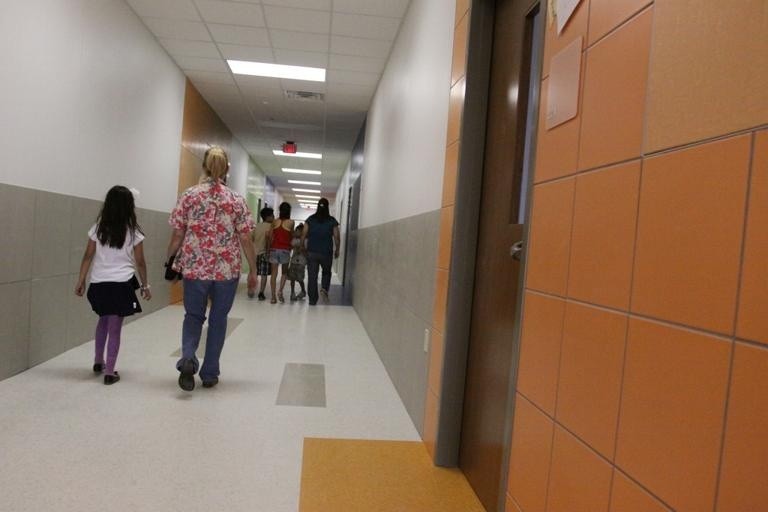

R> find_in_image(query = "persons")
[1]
[248,198,340,305]
[164,145,258,390]
[75,185,152,384]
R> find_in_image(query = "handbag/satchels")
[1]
[164,255,183,280]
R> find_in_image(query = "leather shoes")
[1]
[103,371,120,384]
[94,362,106,372]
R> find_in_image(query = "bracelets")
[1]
[164,262,167,267]
[140,284,151,290]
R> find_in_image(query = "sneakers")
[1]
[179,357,195,391]
[203,377,218,388]
[259,288,329,304]
